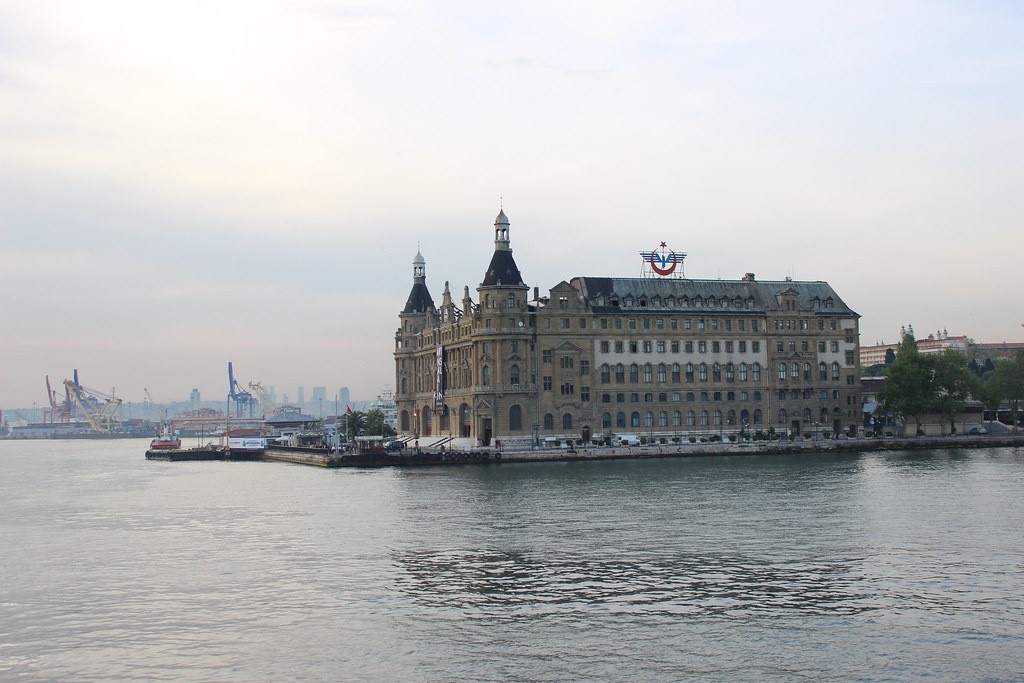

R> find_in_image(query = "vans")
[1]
[612,435,642,449]
[384,441,405,452]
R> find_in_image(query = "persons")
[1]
[310,443,357,455]
[495,439,501,448]
[415,440,420,454]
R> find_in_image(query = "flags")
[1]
[346,405,352,415]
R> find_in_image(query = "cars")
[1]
[969,426,988,434]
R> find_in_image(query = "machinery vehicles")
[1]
[42,361,279,435]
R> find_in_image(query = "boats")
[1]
[148,409,181,450]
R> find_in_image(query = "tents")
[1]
[276,436,292,442]
